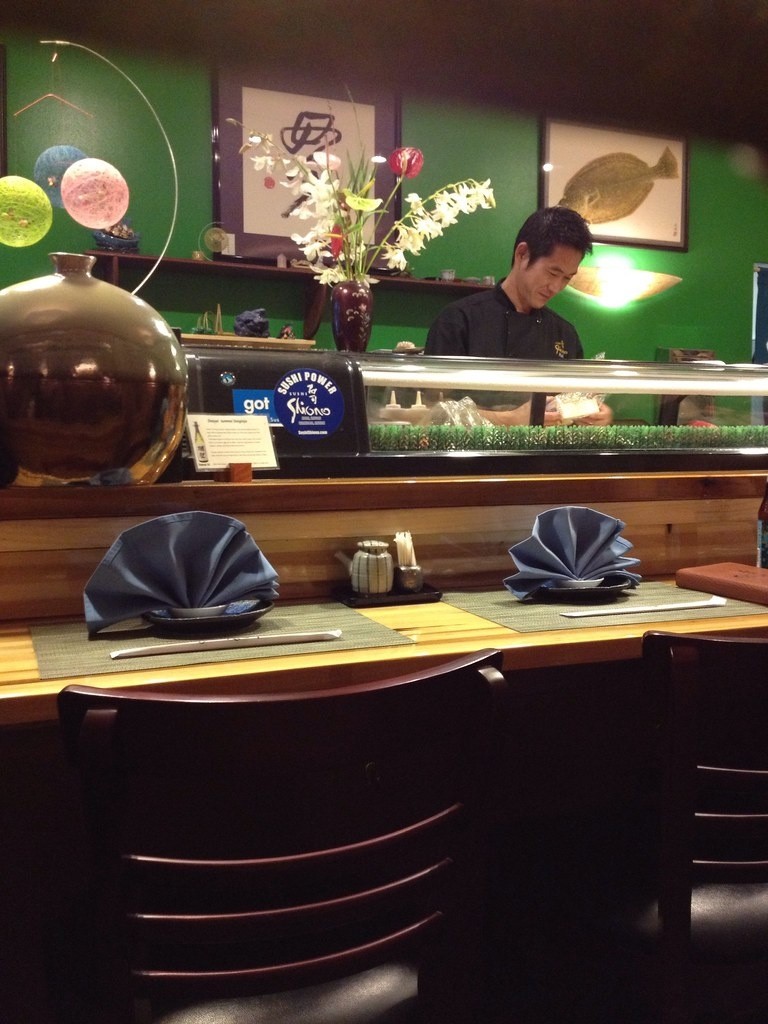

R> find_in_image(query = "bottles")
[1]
[411,390,427,409]
[386,390,401,408]
[438,392,444,401]
[756,483,768,568]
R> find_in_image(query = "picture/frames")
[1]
[537,111,689,254]
[208,52,402,271]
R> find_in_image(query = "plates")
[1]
[557,577,605,587]
[147,601,275,632]
[538,575,631,601]
[169,605,228,616]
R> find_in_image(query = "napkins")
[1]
[501,506,644,601]
[82,511,281,635]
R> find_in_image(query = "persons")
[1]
[407,207,615,426]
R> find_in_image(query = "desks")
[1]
[0,574,768,726]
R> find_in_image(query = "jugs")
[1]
[335,540,394,593]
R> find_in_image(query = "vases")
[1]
[331,281,374,351]
[0,251,189,486]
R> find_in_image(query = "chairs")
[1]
[52,649,510,1024]
[593,636,768,1024]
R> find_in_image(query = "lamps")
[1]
[572,266,683,302]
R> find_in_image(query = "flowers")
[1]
[226,115,498,289]
